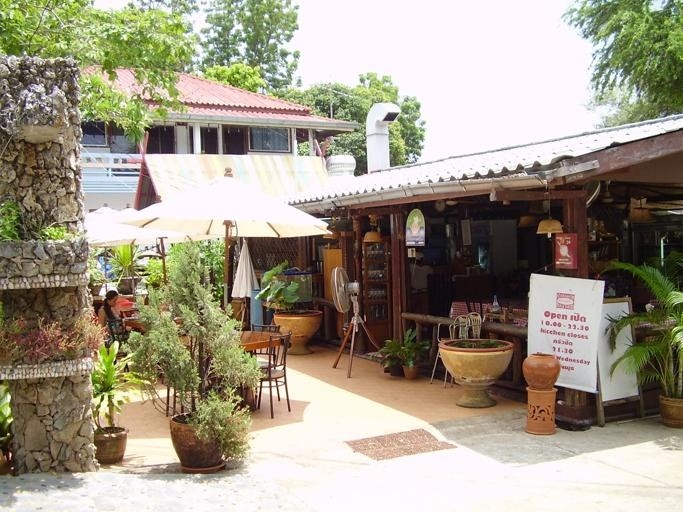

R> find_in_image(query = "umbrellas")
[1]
[87,202,233,286]
[117,166,333,315]
[82,202,119,293]
[230,239,261,309]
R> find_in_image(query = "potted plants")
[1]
[254,259,323,354]
[90,341,152,464]
[380,339,405,377]
[106,244,142,295]
[402,327,430,379]
[127,240,263,474]
[87,249,107,295]
[599,249,683,429]
[335,219,355,237]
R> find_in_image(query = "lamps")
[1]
[536,189,564,240]
[362,216,383,244]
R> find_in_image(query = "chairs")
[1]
[250,323,281,401]
[469,311,482,338]
[429,315,470,389]
[257,334,293,418]
[107,318,130,372]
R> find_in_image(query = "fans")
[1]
[330,267,382,378]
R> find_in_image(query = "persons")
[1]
[97,289,126,337]
[409,251,434,291]
[406,222,424,243]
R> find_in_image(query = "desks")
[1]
[449,299,528,371]
[237,332,282,411]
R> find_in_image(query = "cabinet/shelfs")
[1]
[630,221,683,313]
[353,235,392,351]
[588,239,617,297]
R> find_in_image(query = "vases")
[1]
[439,339,514,407]
[522,353,559,391]
